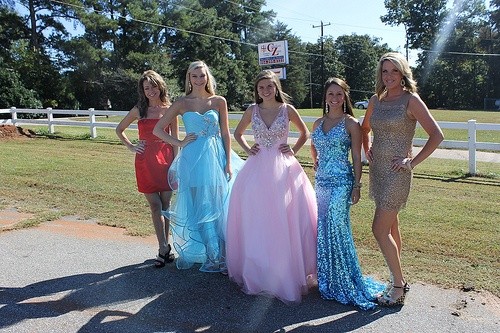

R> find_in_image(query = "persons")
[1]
[310,77,386,311]
[362,52,444,307]
[226,70,318,306]
[153,61,245,275]
[116,70,180,268]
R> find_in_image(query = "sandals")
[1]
[206,252,228,275]
[154,244,175,268]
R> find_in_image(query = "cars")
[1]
[241,101,256,109]
[354,100,369,109]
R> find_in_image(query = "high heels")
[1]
[375,278,410,307]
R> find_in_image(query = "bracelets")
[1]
[407,158,414,170]
[353,182,362,188]
